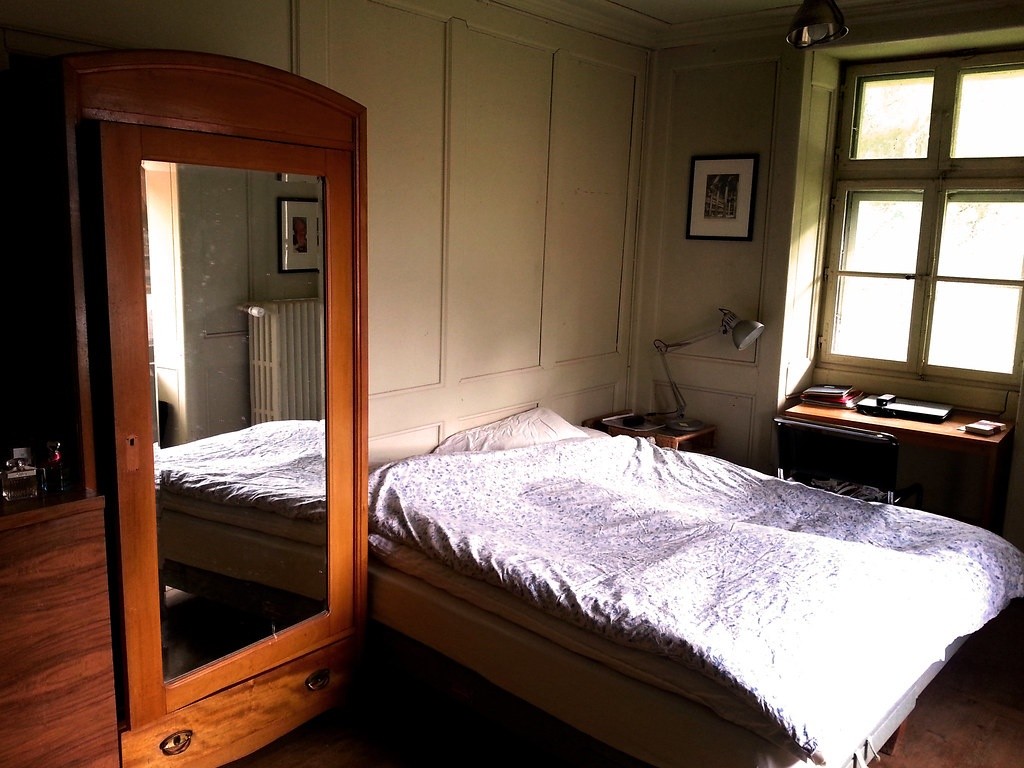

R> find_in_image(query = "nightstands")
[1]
[608,416,719,457]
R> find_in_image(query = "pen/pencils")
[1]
[603,413,634,421]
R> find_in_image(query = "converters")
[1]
[876,394,896,407]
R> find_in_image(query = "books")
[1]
[966,419,1007,436]
[802,384,865,410]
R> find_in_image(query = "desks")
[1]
[0,487,120,768]
[785,405,1014,536]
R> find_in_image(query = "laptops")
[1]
[857,394,954,423]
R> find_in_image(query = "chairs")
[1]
[772,415,924,511]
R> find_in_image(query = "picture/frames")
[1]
[277,194,321,273]
[685,154,759,241]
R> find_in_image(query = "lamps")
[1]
[785,0,850,48]
[652,307,765,430]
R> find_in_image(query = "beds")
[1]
[366,406,1024,768]
[154,419,328,605]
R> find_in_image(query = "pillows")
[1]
[434,407,591,452]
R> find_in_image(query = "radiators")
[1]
[235,296,320,425]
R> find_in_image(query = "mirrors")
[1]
[78,117,358,728]
